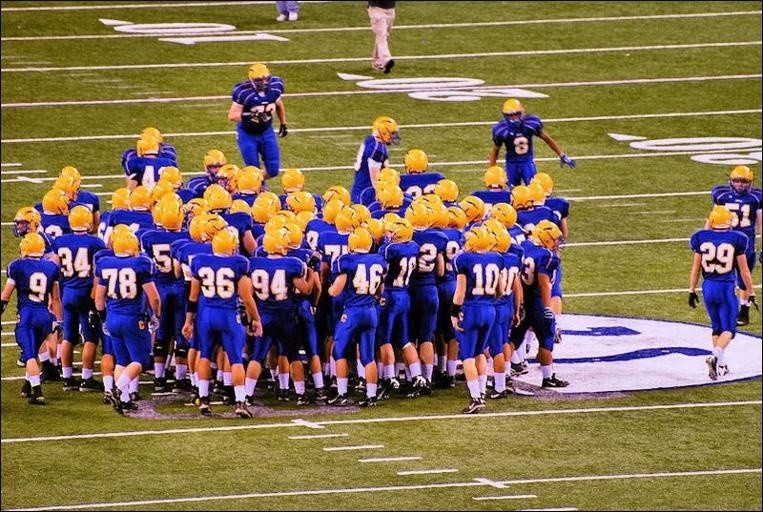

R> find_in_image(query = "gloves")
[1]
[255,112,272,122]
[543,307,553,330]
[148,315,160,333]
[560,152,576,169]
[101,316,111,336]
[689,288,699,308]
[748,295,759,310]
[277,123,288,137]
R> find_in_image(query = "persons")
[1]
[351,115,401,206]
[226,63,290,175]
[271,0,304,24]
[1,148,569,420]
[710,165,762,330]
[366,2,399,77]
[488,98,575,189]
[123,127,178,187]
[685,203,761,381]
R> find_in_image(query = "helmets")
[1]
[322,166,561,254]
[373,117,401,144]
[249,64,272,97]
[13,207,45,257]
[112,166,183,230]
[405,149,427,172]
[730,165,754,181]
[185,150,315,257]
[710,205,733,229]
[501,99,524,127]
[42,167,94,231]
[136,127,161,157]
[110,223,138,258]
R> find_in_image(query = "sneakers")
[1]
[542,372,569,388]
[151,369,254,419]
[101,384,140,413]
[267,374,432,407]
[705,355,717,380]
[433,358,529,398]
[554,321,561,344]
[718,363,730,376]
[462,398,486,414]
[275,12,298,21]
[372,59,394,73]
[735,305,748,325]
[16,352,102,405]
